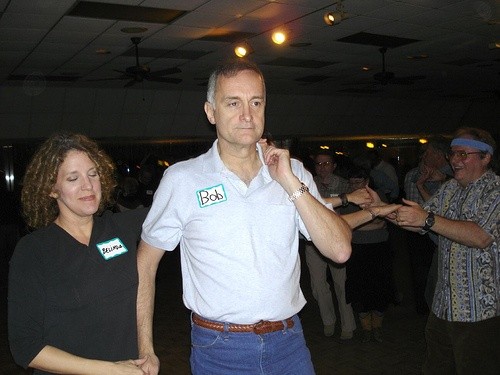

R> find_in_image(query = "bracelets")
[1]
[363,206,375,220]
[339,192,349,206]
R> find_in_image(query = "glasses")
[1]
[349,179,365,186]
[316,161,330,167]
[446,149,490,161]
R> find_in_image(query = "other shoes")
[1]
[340,331,354,340]
[323,323,335,336]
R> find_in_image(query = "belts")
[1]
[191,313,295,334]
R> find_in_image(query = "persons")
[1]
[361,128,500,374]
[7,132,267,375]
[136,57,353,375]
[261,135,456,341]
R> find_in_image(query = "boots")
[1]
[361,329,372,343]
[372,327,385,343]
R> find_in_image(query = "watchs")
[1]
[423,212,435,231]
[288,182,310,203]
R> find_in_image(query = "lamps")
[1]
[234,40,252,58]
[271,28,291,45]
[322,0,352,27]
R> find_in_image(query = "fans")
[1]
[85,36,182,90]
[352,44,426,87]
